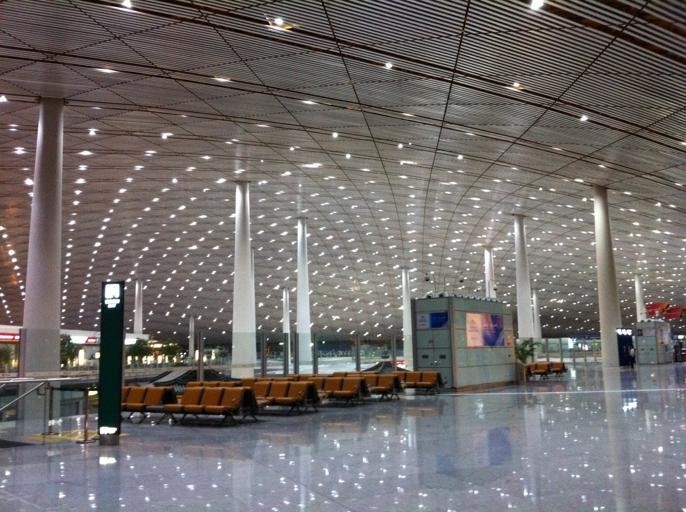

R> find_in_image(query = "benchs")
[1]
[333,372,445,396]
[255,371,402,408]
[122,380,319,427]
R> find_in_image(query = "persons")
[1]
[622,344,636,368]
[674,342,681,362]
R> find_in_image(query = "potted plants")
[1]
[515,336,544,384]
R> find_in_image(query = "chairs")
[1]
[526,361,571,380]
